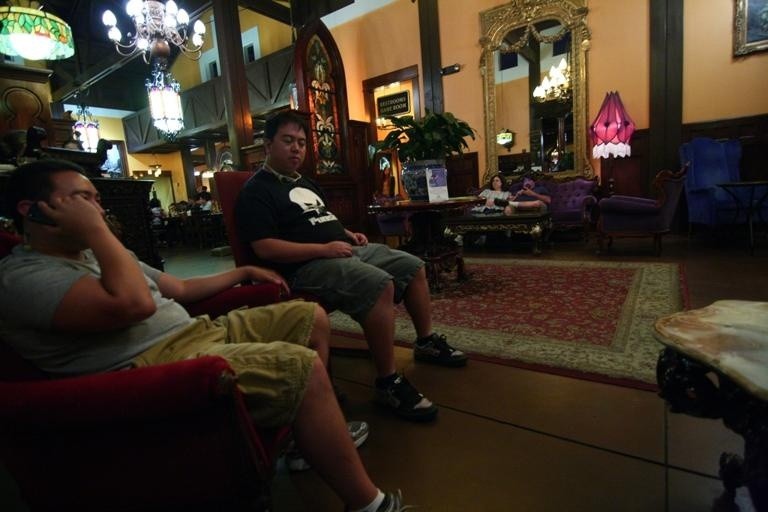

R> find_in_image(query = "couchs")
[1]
[679,138,768,239]
[465,169,598,243]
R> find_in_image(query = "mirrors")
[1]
[479,1,593,186]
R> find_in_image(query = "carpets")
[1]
[328,256,691,393]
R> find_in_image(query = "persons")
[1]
[378,165,395,198]
[0,161,406,510]
[233,113,467,422]
[504,176,551,215]
[468,176,512,211]
[188,183,214,212]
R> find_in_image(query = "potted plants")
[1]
[375,107,475,201]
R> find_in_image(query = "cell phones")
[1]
[25,202,57,228]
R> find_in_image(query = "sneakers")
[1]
[342,486,424,512]
[494,197,509,208]
[371,368,440,425]
[341,419,371,449]
[412,332,470,368]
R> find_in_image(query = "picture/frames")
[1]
[733,0,768,59]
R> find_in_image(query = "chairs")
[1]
[598,161,691,258]
[215,170,372,413]
[0,232,291,511]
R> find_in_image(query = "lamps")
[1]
[1,0,75,61]
[72,87,99,153]
[146,56,187,143]
[496,128,513,147]
[102,0,206,66]
[590,91,636,196]
[532,58,572,105]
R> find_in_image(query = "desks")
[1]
[653,299,768,511]
[442,210,551,256]
[716,180,768,257]
[368,198,486,293]
